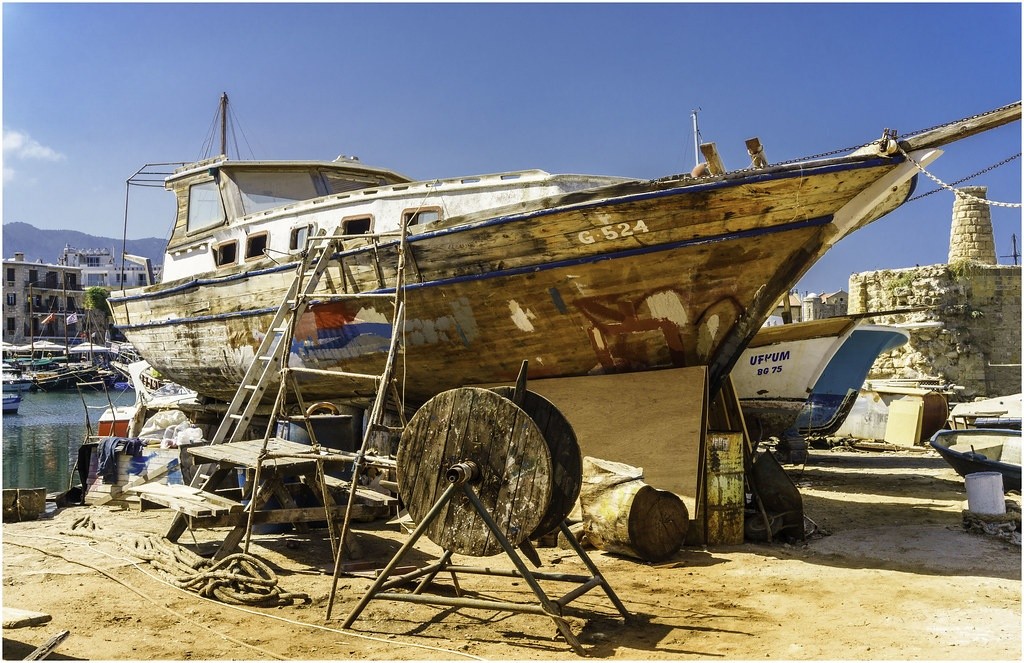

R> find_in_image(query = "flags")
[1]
[39,312,80,326]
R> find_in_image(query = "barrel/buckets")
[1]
[964,471,1006,514]
[236,466,296,536]
[276,400,356,526]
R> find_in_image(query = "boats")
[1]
[732,306,928,466]
[930,392,1022,495]
[3,255,197,439]
[100,91,1023,561]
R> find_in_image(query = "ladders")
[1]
[174,223,343,543]
